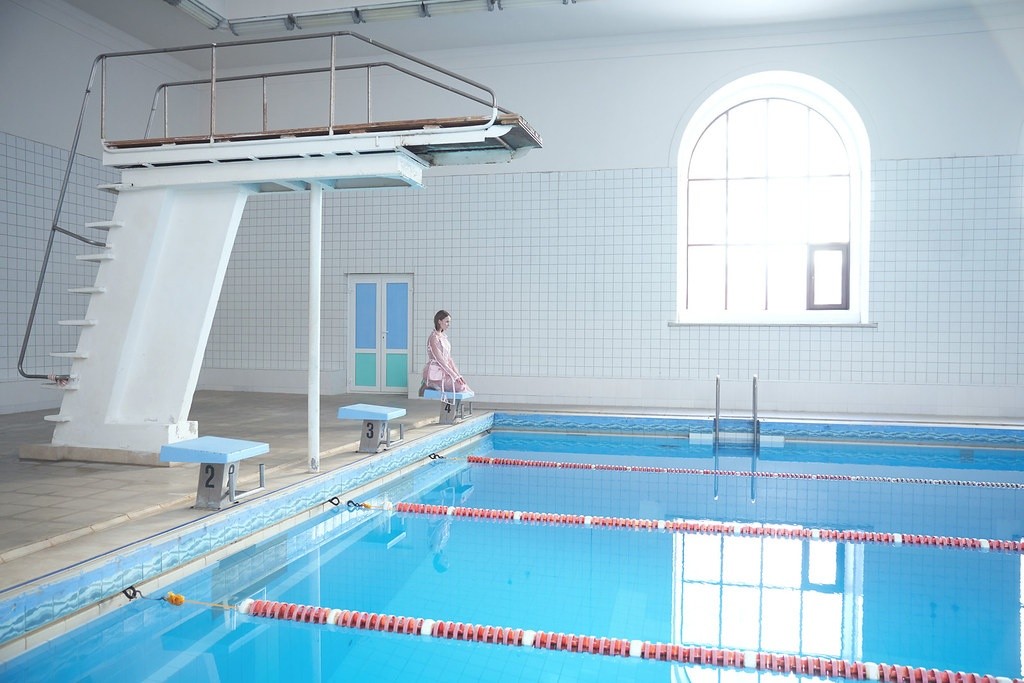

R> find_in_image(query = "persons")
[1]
[418,310,467,398]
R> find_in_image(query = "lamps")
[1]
[228,14,294,36]
[292,7,360,30]
[167,0,225,30]
[422,0,504,17]
[498,0,569,11]
[357,1,426,23]
